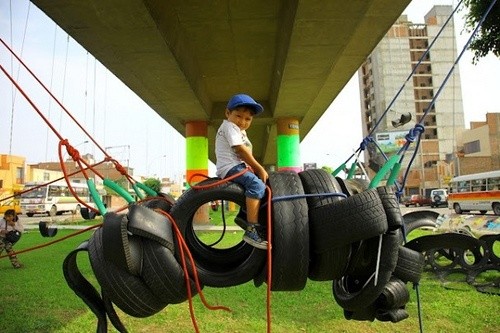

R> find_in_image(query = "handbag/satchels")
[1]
[7,229,21,243]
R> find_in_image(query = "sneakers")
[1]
[234,208,248,229]
[243,224,272,249]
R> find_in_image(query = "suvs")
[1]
[430,189,448,208]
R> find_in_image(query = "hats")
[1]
[228,95,263,113]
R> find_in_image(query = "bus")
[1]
[445,170,500,215]
[19,180,103,217]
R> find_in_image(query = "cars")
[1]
[402,193,431,207]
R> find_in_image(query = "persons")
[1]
[0,210,24,268]
[215,94,272,250]
